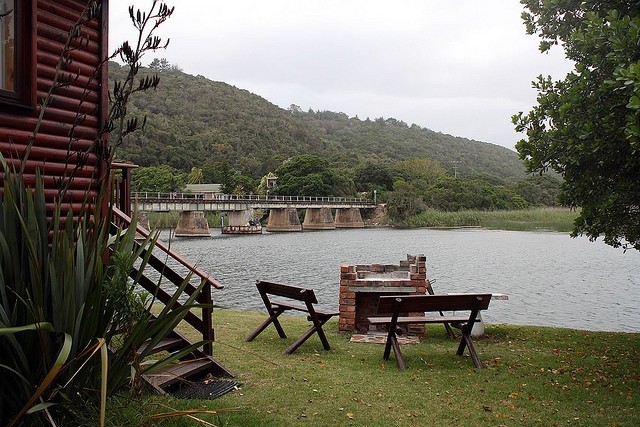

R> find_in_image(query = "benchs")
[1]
[367,294,492,373]
[244,278,340,356]
